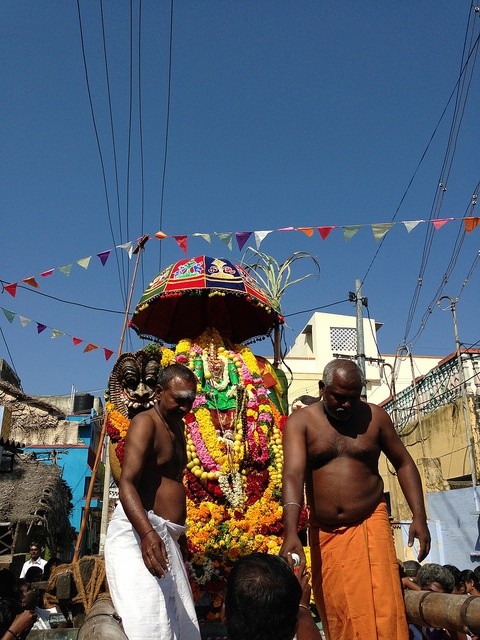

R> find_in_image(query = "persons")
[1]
[0,542,63,640]
[107,349,162,420]
[396,558,480,640]
[222,552,323,640]
[279,360,431,640]
[105,364,201,640]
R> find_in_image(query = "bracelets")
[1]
[282,502,302,510]
[7,629,19,640]
[139,529,156,541]
[298,604,312,613]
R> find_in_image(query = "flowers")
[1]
[103,325,316,604]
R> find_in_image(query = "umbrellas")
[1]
[128,255,286,348]
